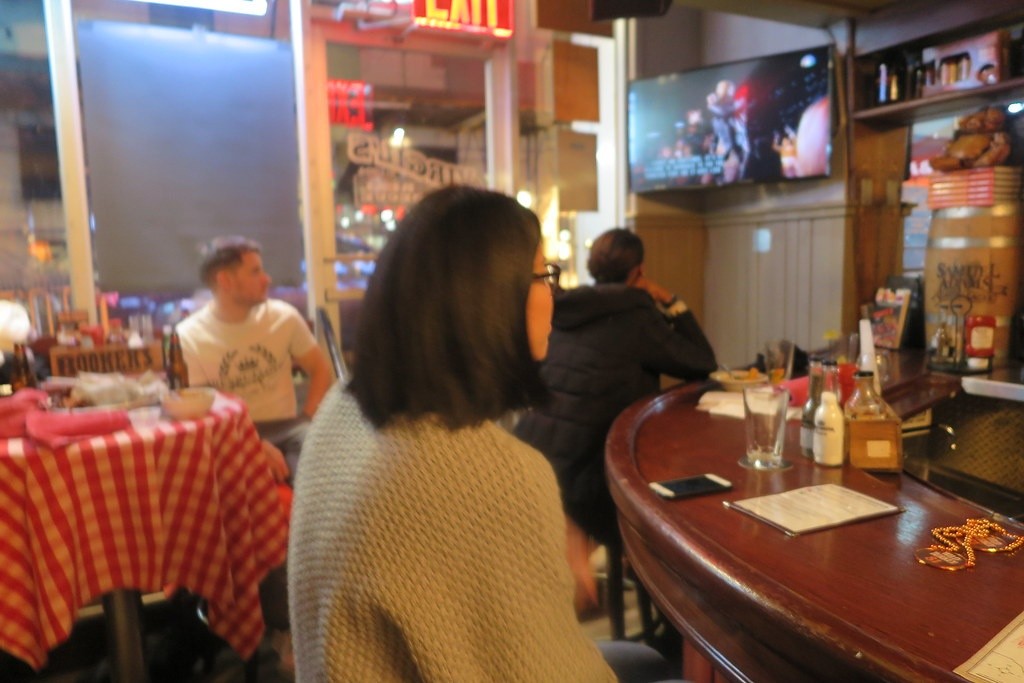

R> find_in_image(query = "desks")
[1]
[0,390,290,683]
[602,338,1024,682]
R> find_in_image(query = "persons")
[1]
[177,242,335,488]
[288,186,689,683]
[515,228,719,548]
[636,80,828,186]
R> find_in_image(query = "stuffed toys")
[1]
[928,110,1009,173]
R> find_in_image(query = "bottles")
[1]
[80,327,94,349]
[127,331,143,348]
[11,341,39,394]
[799,353,844,465]
[935,327,949,359]
[161,325,189,393]
[844,369,887,420]
[965,316,995,370]
[108,318,125,344]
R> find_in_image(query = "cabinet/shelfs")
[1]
[842,8,1024,341]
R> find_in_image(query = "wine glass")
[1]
[763,337,797,403]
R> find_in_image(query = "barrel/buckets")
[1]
[923,201,1024,366]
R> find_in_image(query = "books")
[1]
[871,285,912,349]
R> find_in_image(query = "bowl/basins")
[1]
[709,368,769,393]
[127,406,162,433]
[160,386,217,421]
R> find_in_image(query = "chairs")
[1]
[8,341,149,393]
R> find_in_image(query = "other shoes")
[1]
[622,559,638,590]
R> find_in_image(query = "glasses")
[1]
[529,262,560,290]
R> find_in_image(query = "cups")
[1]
[741,384,790,467]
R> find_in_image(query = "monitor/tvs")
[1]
[626,44,833,194]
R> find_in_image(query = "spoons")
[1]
[719,362,744,381]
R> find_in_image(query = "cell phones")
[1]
[649,473,733,498]
